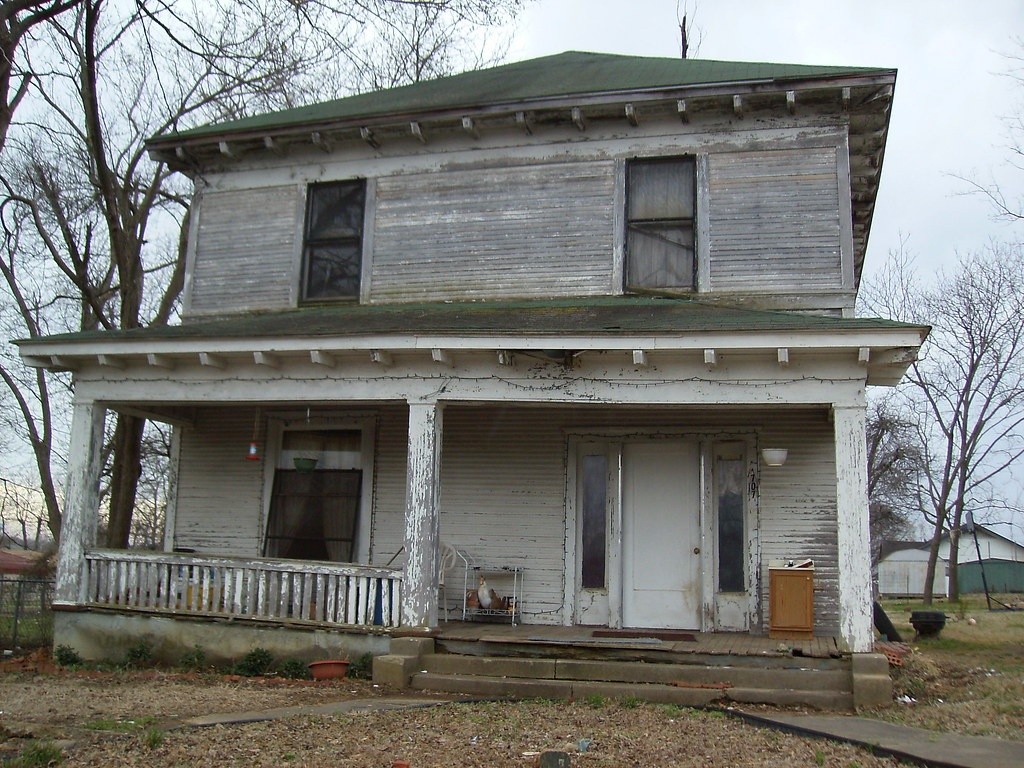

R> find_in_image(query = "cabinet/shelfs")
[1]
[452,543,525,628]
[768,570,815,640]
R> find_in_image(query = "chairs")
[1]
[438,540,457,625]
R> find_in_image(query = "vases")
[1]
[293,457,318,475]
[308,658,353,680]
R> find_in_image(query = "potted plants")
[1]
[753,437,790,468]
